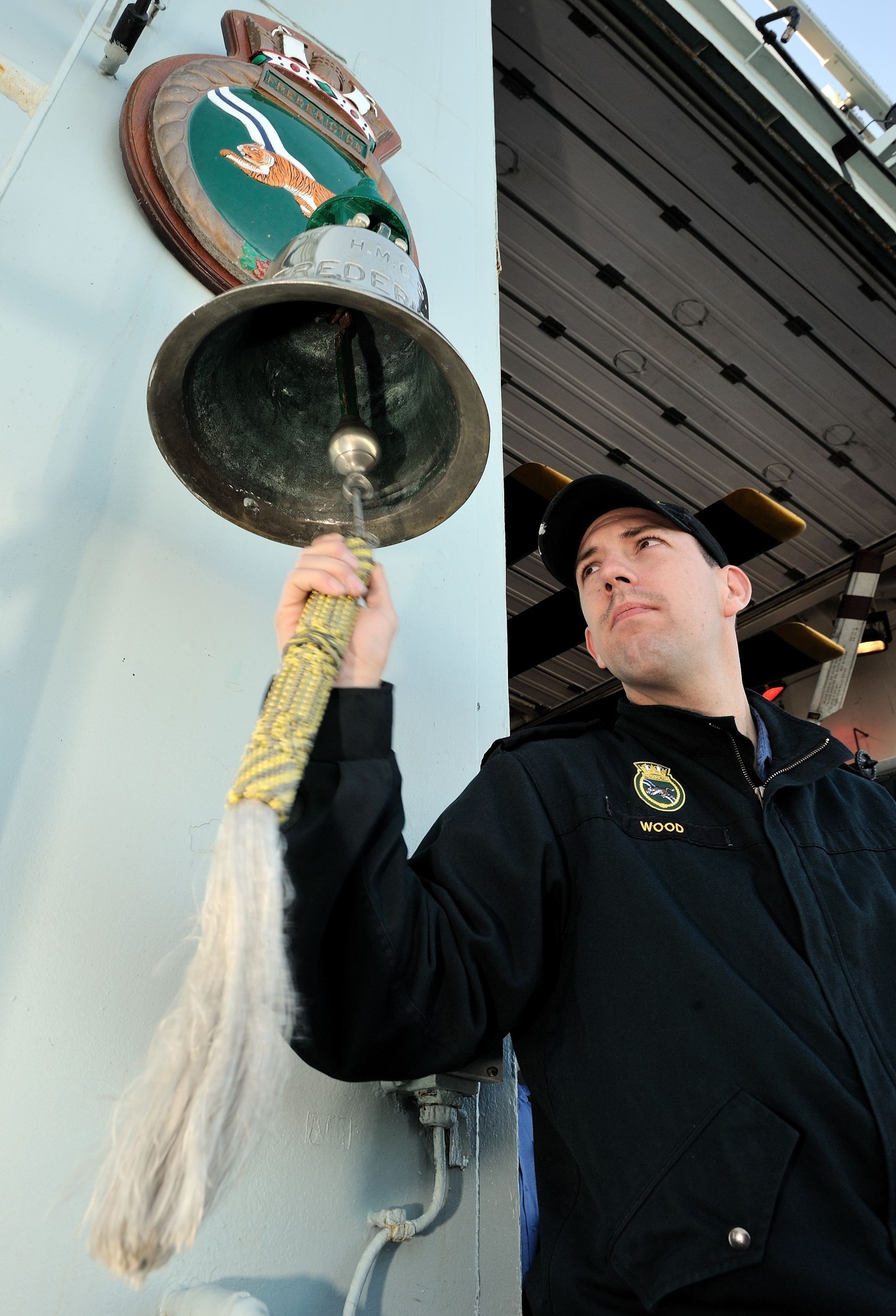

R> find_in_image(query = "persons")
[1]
[265,471,895,1316]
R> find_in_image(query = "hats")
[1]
[537,473,731,596]
